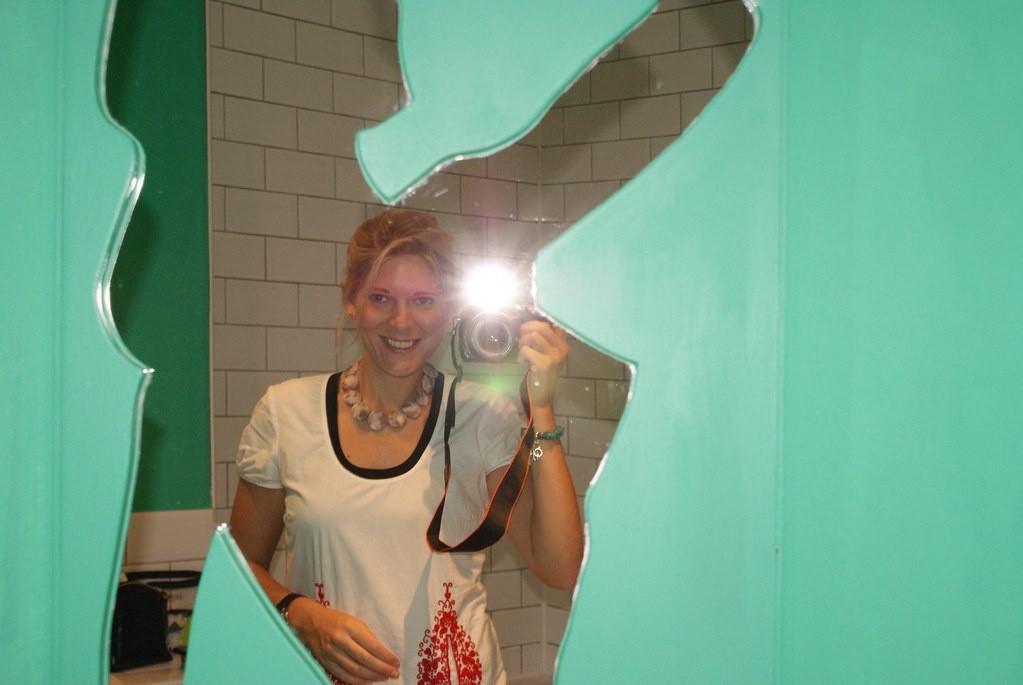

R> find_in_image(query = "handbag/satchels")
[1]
[110,582,172,673]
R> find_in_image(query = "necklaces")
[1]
[344,355,438,431]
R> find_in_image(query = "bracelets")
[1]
[530,425,564,465]
[276,592,309,625]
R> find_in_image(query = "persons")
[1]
[228,208,583,685]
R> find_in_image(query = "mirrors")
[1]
[95,0,767,685]
